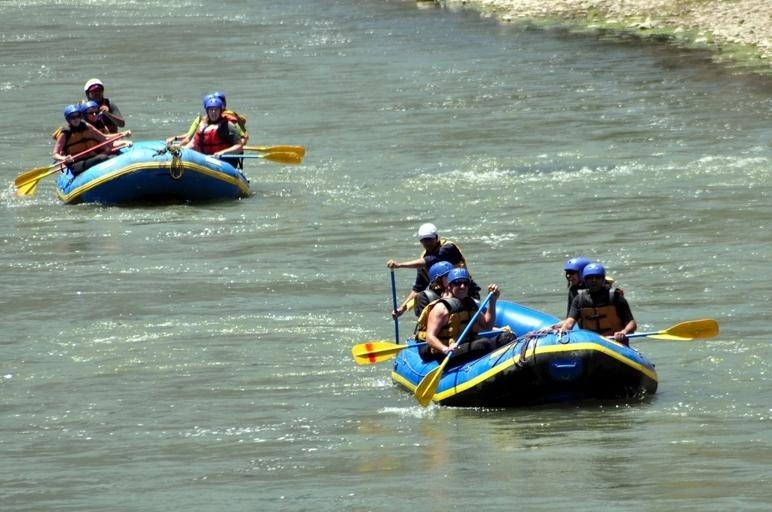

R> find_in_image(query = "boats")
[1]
[392,295,659,411]
[56,140,254,205]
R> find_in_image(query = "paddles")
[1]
[414,287,498,408]
[606,318,719,341]
[352,328,511,365]
[14,131,129,198]
[220,146,304,164]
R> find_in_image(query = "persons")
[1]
[388,223,480,318]
[552,264,637,348]
[564,257,618,317]
[414,262,452,318]
[168,93,248,168]
[425,267,515,367]
[52,78,124,175]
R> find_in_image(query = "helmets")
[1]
[203,92,226,109]
[429,261,471,285]
[85,78,103,92]
[64,100,98,117]
[564,258,604,280]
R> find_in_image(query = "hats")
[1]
[418,223,437,240]
